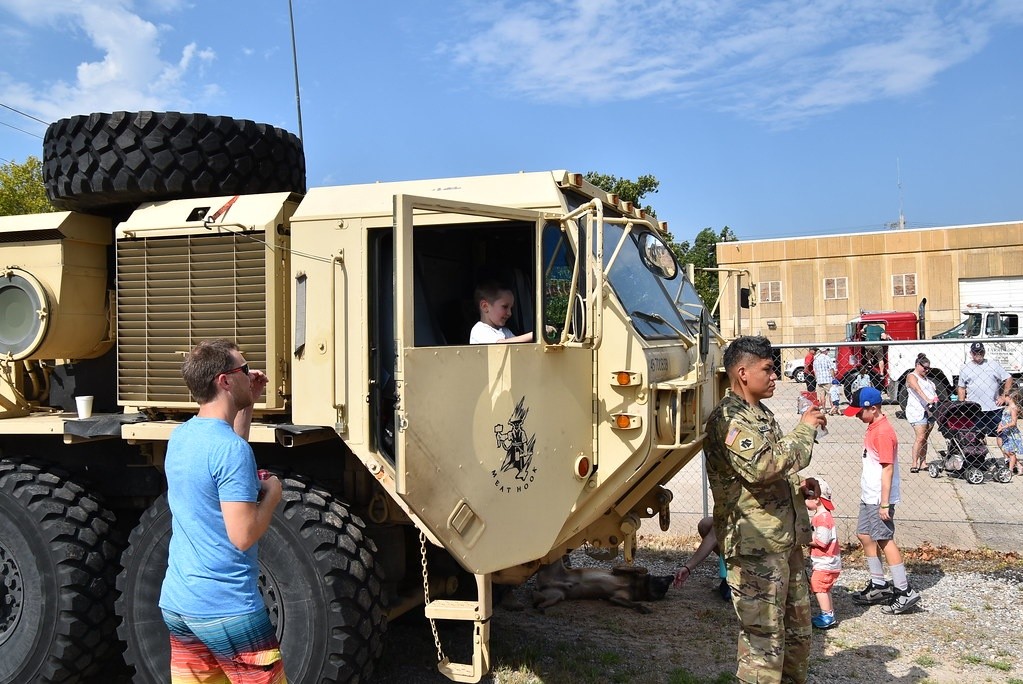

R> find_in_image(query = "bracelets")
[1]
[684,566,691,574]
[880,503,890,508]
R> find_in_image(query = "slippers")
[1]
[910,467,919,473]
[918,466,929,471]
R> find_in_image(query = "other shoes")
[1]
[867,363,872,367]
[1004,462,1023,476]
[822,409,844,416]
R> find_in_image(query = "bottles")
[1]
[797,395,828,439]
[950,394,958,402]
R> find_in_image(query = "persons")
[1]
[843,387,921,615]
[905,353,939,473]
[804,323,887,416]
[958,342,1018,473]
[470,279,558,344]
[672,517,731,601]
[158,339,289,684]
[800,476,842,628]
[702,335,827,684]
[997,388,1023,476]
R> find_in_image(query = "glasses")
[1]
[214,362,249,379]
[919,362,930,370]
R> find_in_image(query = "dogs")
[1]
[534,555,675,615]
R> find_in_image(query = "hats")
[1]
[971,342,985,352]
[832,379,839,385]
[809,347,819,350]
[878,373,884,379]
[843,386,882,417]
[819,347,830,351]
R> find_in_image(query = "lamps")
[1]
[767,320,775,329]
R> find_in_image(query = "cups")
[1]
[75,396,94,419]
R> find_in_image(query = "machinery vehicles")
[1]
[836,298,1023,419]
[0,108,757,684]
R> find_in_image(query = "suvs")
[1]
[784,346,835,383]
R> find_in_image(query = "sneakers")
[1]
[880,582,921,615]
[811,609,836,628]
[852,578,894,605]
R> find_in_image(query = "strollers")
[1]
[925,399,1012,484]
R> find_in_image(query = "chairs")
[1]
[512,294,532,331]
[438,299,468,345]
